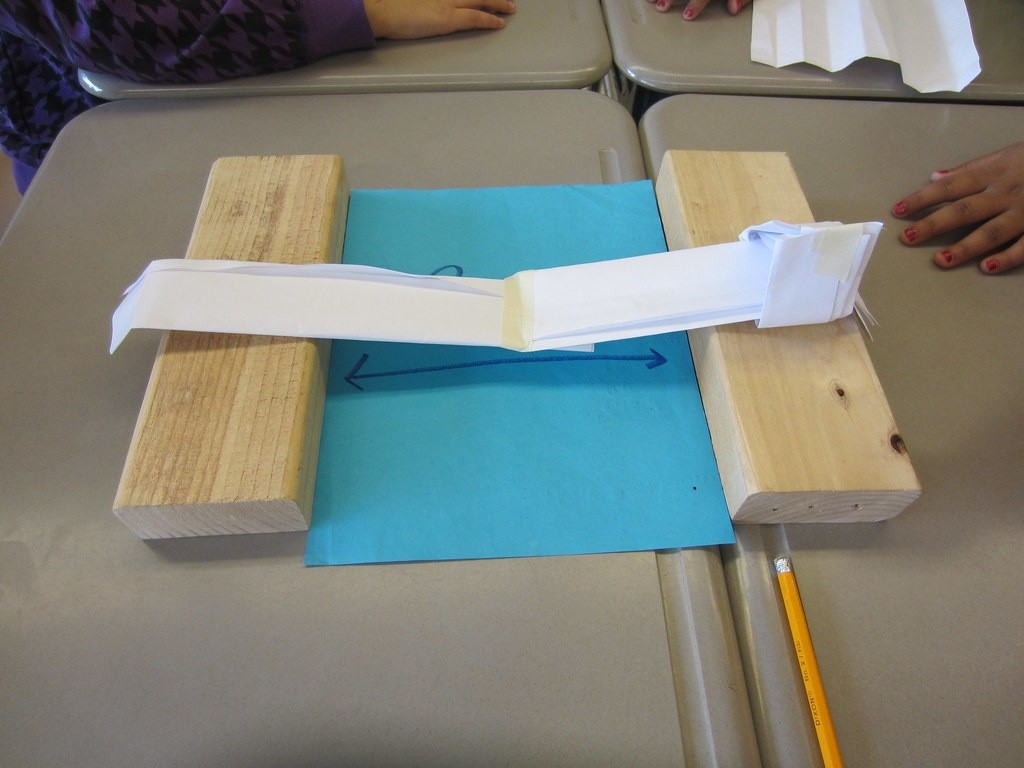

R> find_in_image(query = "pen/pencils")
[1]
[772,555,847,768]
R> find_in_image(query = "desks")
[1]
[0,0,1024,768]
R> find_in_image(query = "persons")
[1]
[889,140,1023,275]
[650,0,748,19]
[0,0,516,198]
